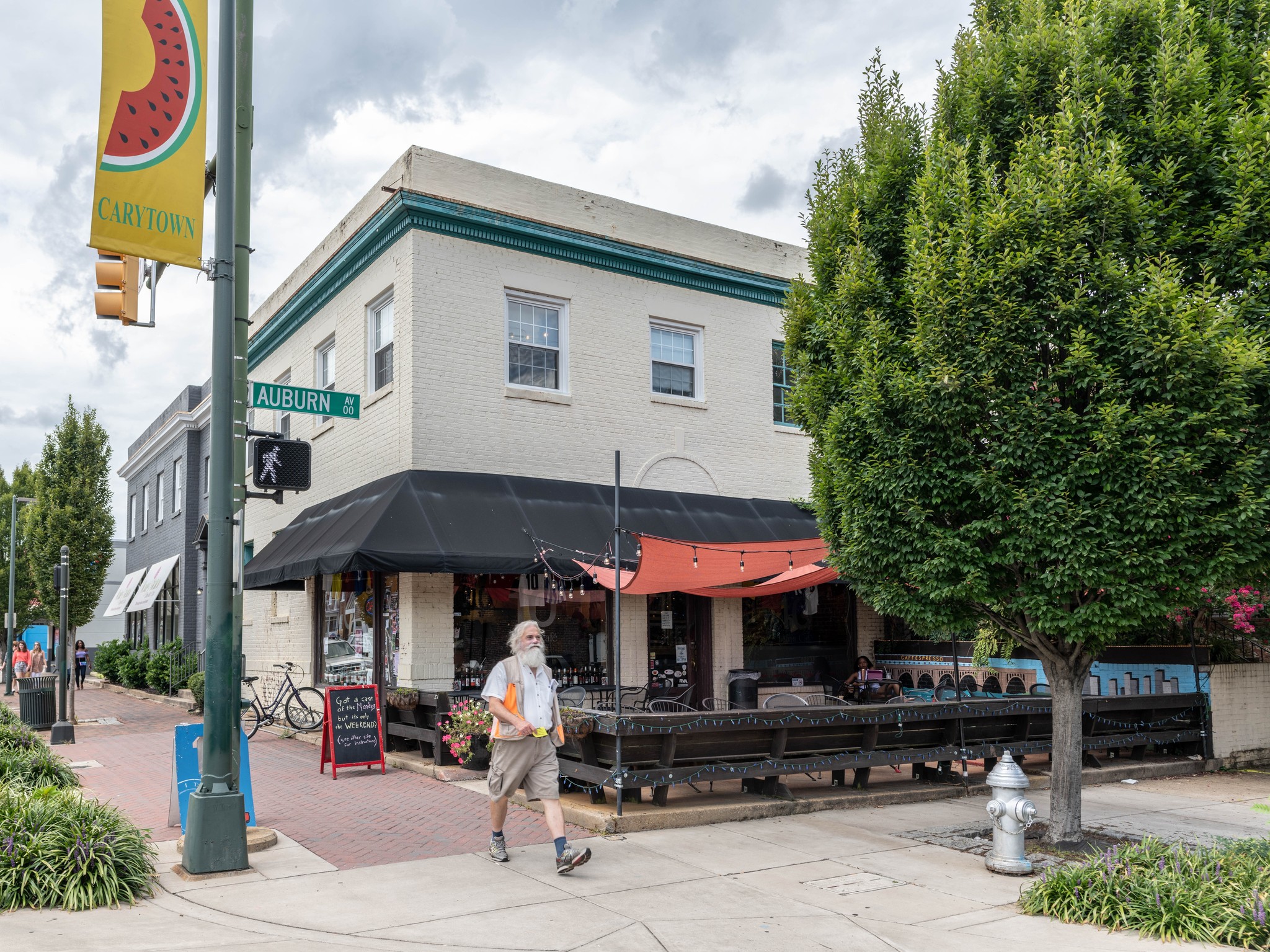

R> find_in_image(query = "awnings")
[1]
[572,531,836,594]
[236,470,845,591]
[604,561,845,599]
[125,554,181,612]
[102,567,148,617]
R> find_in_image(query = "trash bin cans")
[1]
[15,676,58,732]
[728,669,759,710]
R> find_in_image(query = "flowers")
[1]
[437,700,496,764]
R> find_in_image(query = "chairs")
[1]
[560,678,1051,713]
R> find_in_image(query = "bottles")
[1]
[464,668,470,690]
[454,667,458,681]
[597,666,603,685]
[485,670,489,684]
[469,667,475,690]
[557,668,562,688]
[573,668,578,685]
[552,666,558,688]
[458,667,465,690]
[324,666,373,687]
[562,668,568,688]
[578,668,583,685]
[480,669,485,690]
[587,666,593,685]
[475,667,480,690]
[568,667,573,688]
[592,667,598,685]
[583,666,588,685]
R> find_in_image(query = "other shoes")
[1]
[16,691,19,694]
[11,689,15,693]
[67,684,69,690]
[80,684,83,689]
[76,688,79,690]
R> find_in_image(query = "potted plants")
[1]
[387,687,419,710]
[560,706,594,739]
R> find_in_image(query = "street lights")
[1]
[6,494,39,696]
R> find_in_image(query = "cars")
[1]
[322,637,374,686]
[348,635,369,657]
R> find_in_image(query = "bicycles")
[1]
[237,662,325,740]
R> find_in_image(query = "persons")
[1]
[844,656,915,703]
[479,620,591,874]
[75,640,92,690]
[0,641,18,693]
[12,641,32,694]
[67,636,72,690]
[29,641,48,689]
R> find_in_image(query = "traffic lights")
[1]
[96,250,143,327]
[253,435,311,493]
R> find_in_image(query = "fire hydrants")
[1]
[984,749,1040,875]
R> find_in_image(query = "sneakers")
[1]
[556,842,592,873]
[488,836,510,862]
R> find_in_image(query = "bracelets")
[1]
[28,666,30,668]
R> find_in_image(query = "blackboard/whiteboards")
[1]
[321,684,384,768]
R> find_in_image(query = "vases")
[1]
[456,733,491,770]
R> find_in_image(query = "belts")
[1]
[526,727,552,740]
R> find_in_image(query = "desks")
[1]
[469,694,484,707]
[573,685,637,710]
[447,690,482,711]
[1002,695,1051,698]
[946,696,1001,701]
[854,679,902,705]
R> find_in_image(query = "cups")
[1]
[860,669,866,680]
[463,665,468,667]
[480,665,485,669]
[470,660,476,670]
[885,673,892,680]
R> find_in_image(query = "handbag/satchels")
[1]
[79,662,87,666]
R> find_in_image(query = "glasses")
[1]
[14,645,18,647]
[34,644,39,646]
